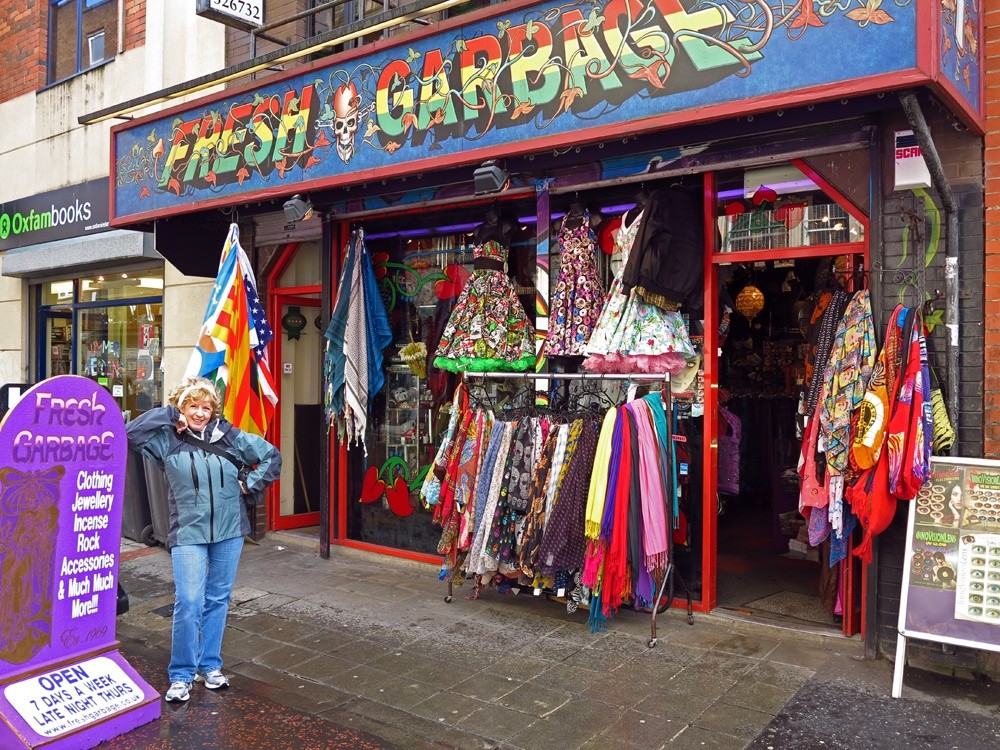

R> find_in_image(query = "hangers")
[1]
[460,371,667,425]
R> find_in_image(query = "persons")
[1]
[543,198,609,359]
[125,376,282,700]
[581,189,696,377]
[434,206,538,374]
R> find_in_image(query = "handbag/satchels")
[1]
[168,425,254,482]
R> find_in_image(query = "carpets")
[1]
[739,590,843,627]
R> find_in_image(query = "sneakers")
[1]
[165,679,193,701]
[194,669,229,690]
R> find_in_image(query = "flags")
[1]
[183,222,279,440]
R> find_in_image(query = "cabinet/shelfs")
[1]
[386,367,434,482]
[51,326,108,362]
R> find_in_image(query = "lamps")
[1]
[474,159,511,195]
[734,262,764,328]
[283,194,314,223]
